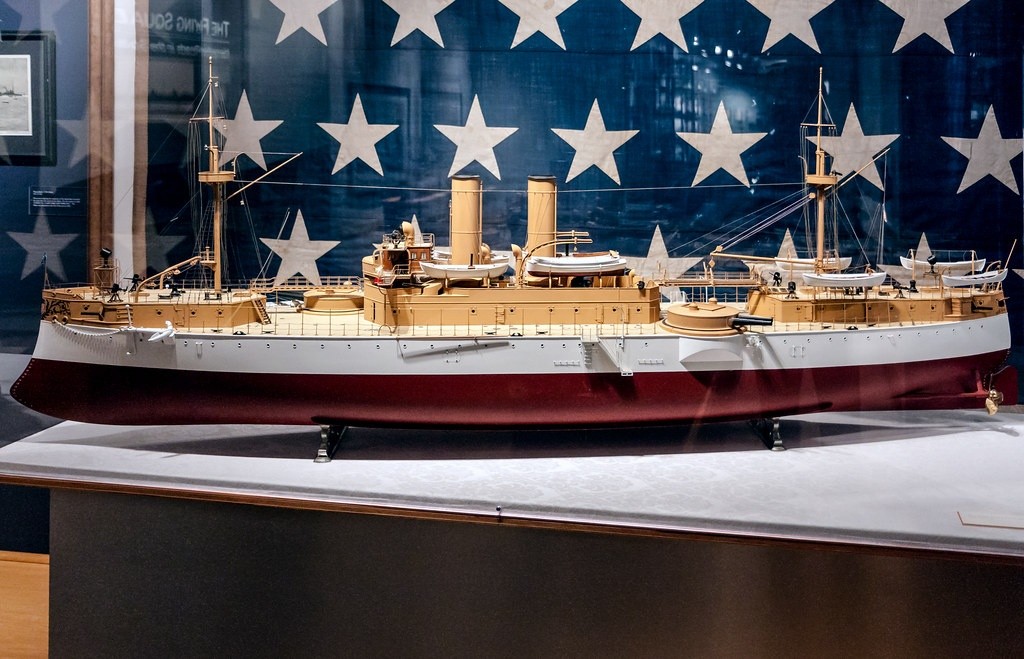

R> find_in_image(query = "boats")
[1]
[6,54,1016,426]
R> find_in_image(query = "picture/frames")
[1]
[0,30,56,166]
